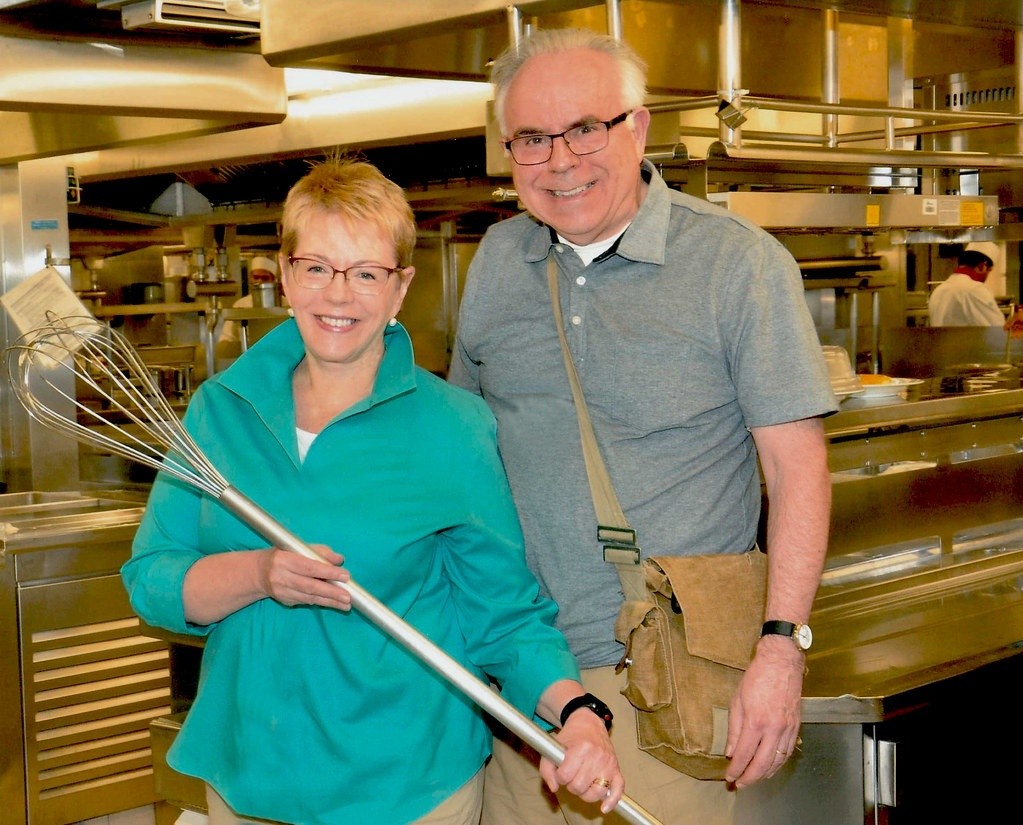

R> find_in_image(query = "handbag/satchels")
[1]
[614,551,807,782]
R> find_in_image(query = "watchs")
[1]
[759,620,812,652]
[560,694,613,731]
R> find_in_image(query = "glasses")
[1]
[285,257,399,295]
[505,109,635,165]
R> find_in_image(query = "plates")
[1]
[830,387,865,404]
[843,378,926,399]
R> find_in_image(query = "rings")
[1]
[594,779,610,788]
[777,750,786,755]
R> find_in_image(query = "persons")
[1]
[928,241,1006,326]
[122,162,624,825]
[444,28,841,825]
[218,254,278,341]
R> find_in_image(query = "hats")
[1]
[966,241,999,265]
[250,256,278,277]
